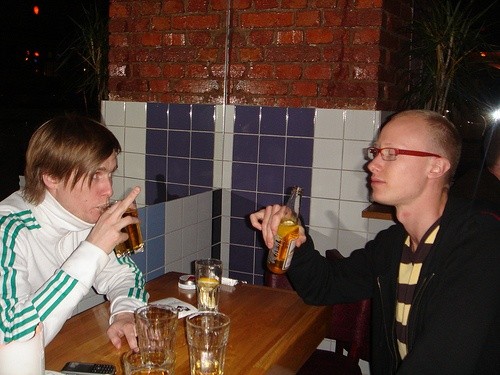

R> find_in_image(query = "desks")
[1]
[45,271,341,375]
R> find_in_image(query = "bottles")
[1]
[266,185,302,275]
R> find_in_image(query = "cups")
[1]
[186,312,231,375]
[135,304,179,367]
[99,197,145,258]
[192,258,224,312]
[122,347,177,374]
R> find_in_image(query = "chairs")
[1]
[263,255,371,375]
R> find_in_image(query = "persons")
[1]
[460,126,500,213]
[0,114,151,352]
[250,109,500,375]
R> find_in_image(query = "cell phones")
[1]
[61,361,118,375]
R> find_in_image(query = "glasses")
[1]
[367,147,443,161]
